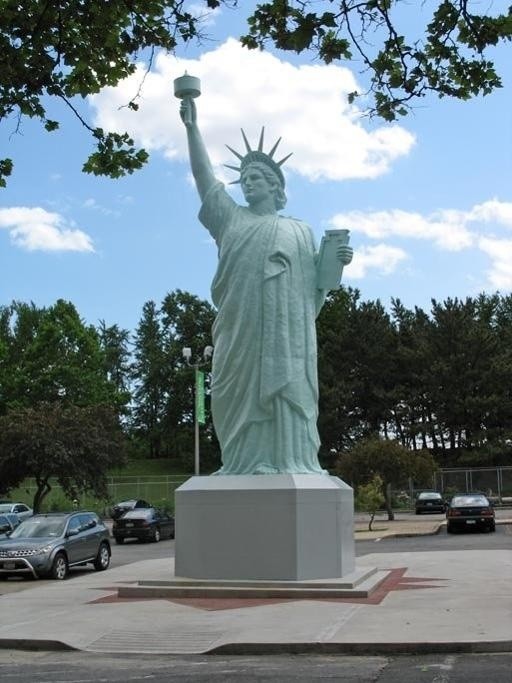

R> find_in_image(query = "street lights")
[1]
[182,345,213,475]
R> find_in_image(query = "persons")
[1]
[180,91,355,477]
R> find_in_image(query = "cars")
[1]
[415,491,446,514]
[0,498,174,580]
[446,493,494,533]
